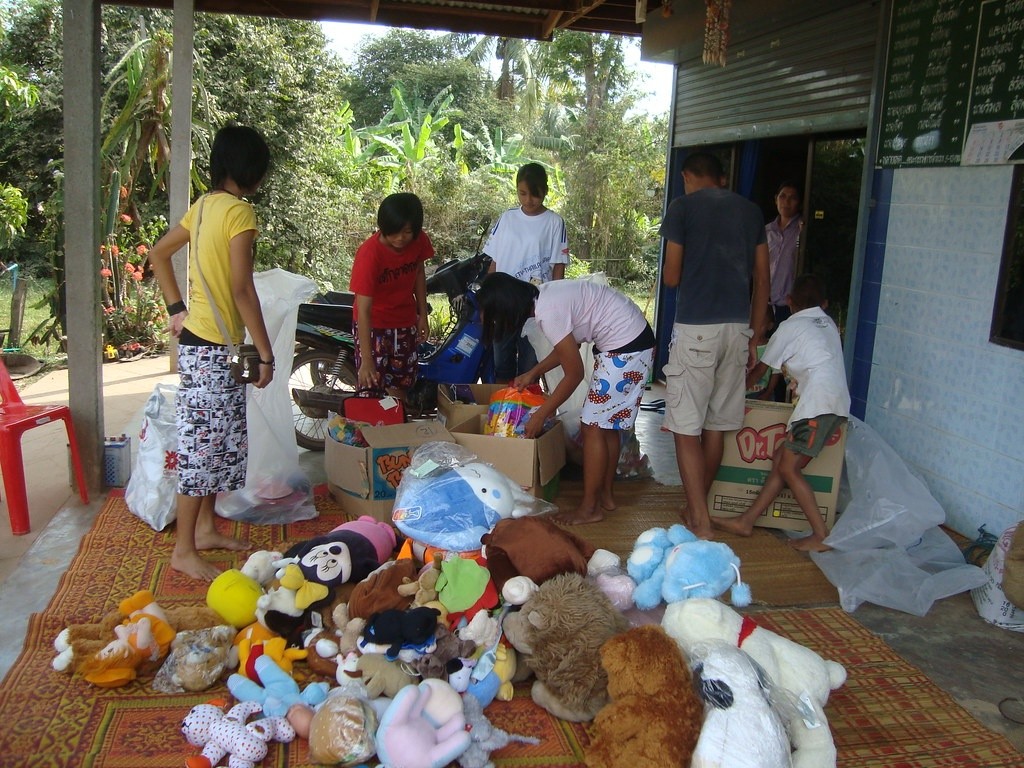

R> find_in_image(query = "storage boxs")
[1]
[446,415,568,507]
[433,379,512,426]
[323,415,454,523]
[705,395,846,532]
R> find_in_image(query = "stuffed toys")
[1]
[52,460,848,768]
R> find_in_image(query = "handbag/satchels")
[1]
[124,383,179,532]
[445,384,475,403]
[338,388,409,424]
[228,345,260,383]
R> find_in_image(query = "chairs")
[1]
[0,357,89,538]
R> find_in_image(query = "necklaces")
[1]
[215,184,238,196]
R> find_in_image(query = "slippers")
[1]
[640,398,665,407]
[641,406,666,414]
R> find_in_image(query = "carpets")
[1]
[0,486,1024,768]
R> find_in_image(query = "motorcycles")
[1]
[252,213,539,452]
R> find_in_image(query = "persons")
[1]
[763,181,801,338]
[660,150,772,541]
[488,163,567,281]
[148,124,276,581]
[478,271,656,528]
[349,192,431,411]
[709,273,849,552]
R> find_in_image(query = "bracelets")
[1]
[258,356,275,365]
[167,300,186,315]
